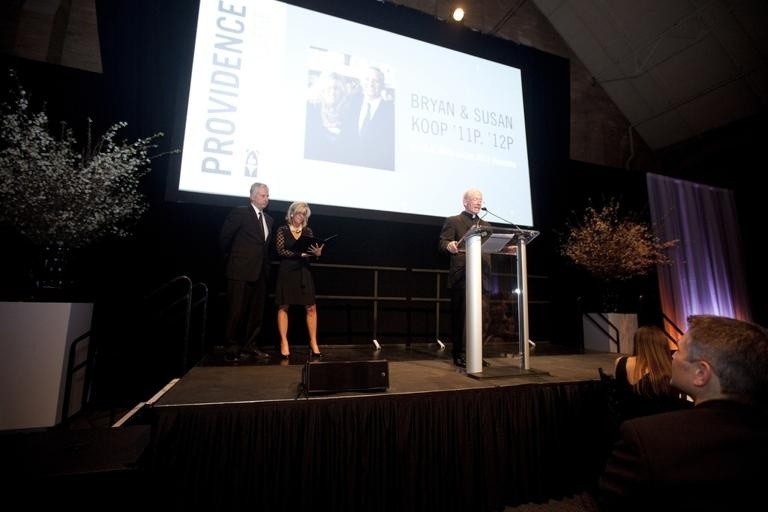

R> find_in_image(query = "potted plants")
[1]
[560,201,679,354]
[0,90,180,429]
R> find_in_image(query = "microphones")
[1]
[482,208,521,229]
[476,208,488,228]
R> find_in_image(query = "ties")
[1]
[259,212,265,243]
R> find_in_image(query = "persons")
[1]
[219,182,275,362]
[440,188,518,368]
[341,67,395,172]
[615,326,674,423]
[276,201,325,361]
[304,72,352,165]
[584,315,768,512]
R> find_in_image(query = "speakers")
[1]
[304,360,389,393]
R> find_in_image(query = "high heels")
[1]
[307,344,327,358]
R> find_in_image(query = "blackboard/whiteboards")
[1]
[176,0,537,229]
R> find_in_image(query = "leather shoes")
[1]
[224,351,241,362]
[241,347,269,361]
[279,343,292,359]
[454,354,490,368]
